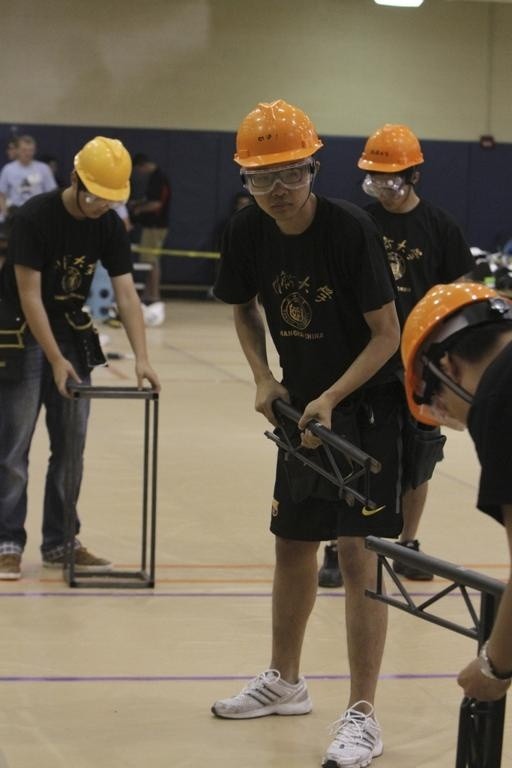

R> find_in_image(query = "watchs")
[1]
[478,640,510,682]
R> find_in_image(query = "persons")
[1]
[401,284,511,702]
[210,98,405,768]
[2,134,163,579]
[237,175,253,211]
[1,133,171,304]
[319,125,479,587]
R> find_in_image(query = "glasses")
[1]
[239,157,316,198]
[360,174,409,200]
[80,188,126,210]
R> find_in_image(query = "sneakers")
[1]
[317,543,345,589]
[321,707,384,768]
[40,537,114,574]
[211,665,312,721]
[392,540,434,582]
[0,541,23,581]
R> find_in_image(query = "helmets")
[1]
[356,122,425,174]
[72,135,134,203]
[399,280,512,427]
[232,96,324,170]
